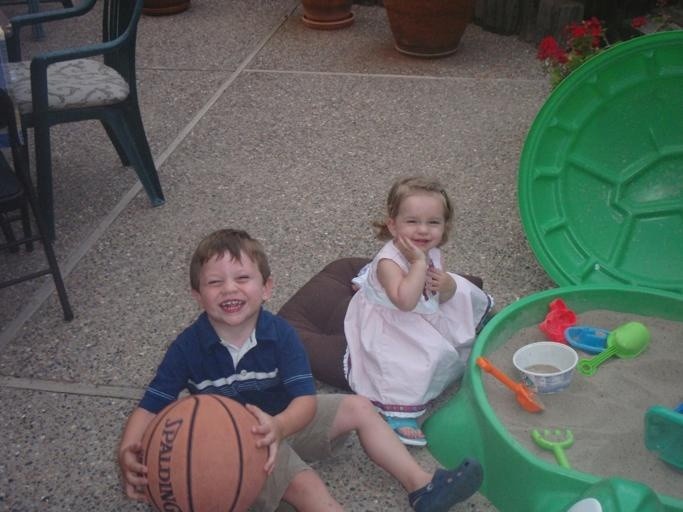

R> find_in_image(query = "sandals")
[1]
[408,458,483,512]
[384,415,428,447]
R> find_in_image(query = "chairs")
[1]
[0,0,165,241]
[0,89,73,323]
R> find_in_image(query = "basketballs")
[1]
[138,393,267,512]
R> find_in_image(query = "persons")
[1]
[116,226,485,512]
[338,175,496,447]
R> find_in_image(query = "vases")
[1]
[142,1,191,15]
[383,0,473,56]
[299,0,355,28]
